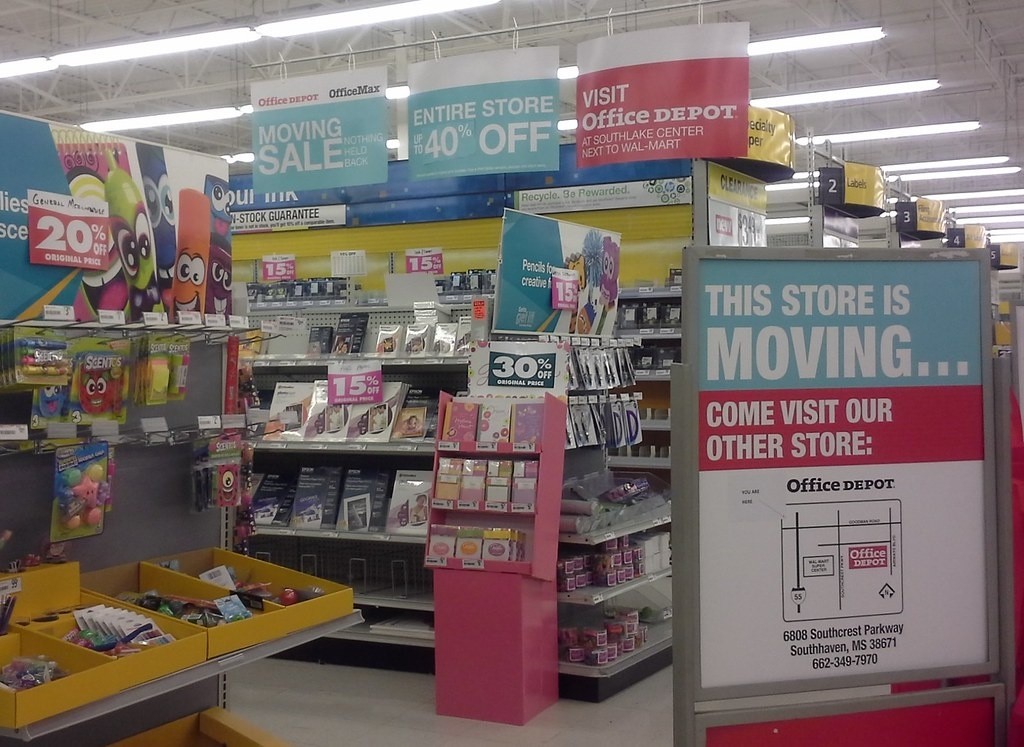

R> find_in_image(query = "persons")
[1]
[336,338,350,354]
[370,405,386,431]
[406,415,418,430]
[326,403,341,430]
[410,494,428,523]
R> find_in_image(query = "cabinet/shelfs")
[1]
[224,107,1016,705]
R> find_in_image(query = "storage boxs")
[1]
[0,546,355,747]
